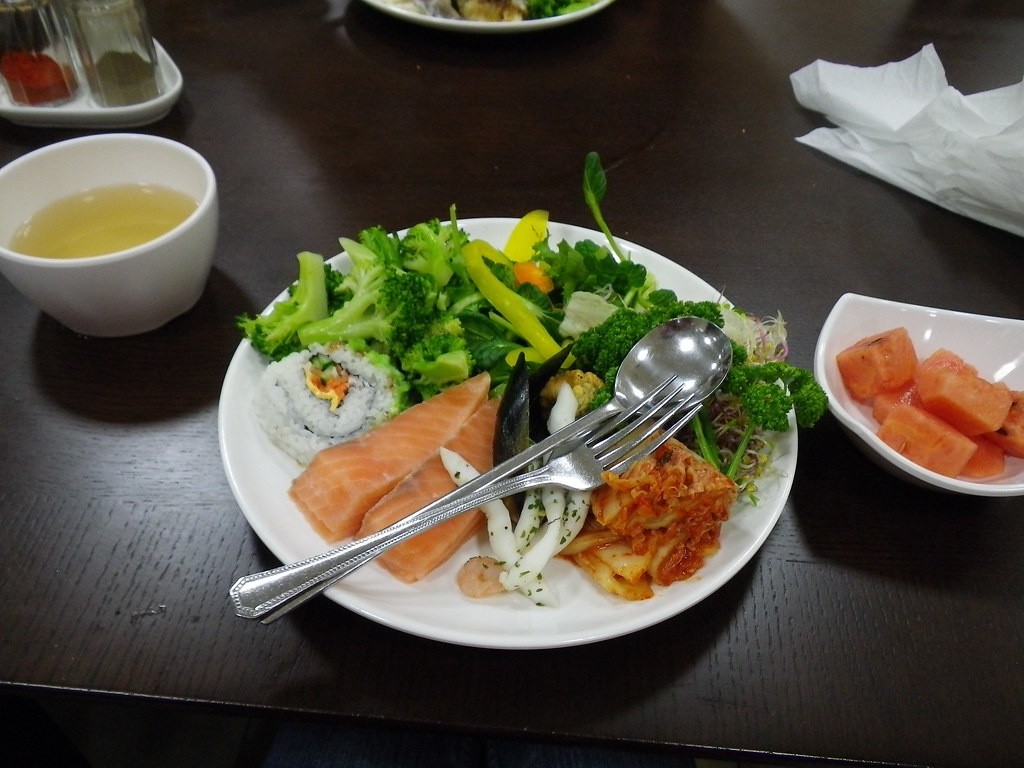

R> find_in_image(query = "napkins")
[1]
[787,43,1024,238]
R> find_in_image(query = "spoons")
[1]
[259,315,733,625]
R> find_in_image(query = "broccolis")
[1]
[240,203,828,480]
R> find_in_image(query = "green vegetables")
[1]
[527,0,596,20]
[525,151,657,310]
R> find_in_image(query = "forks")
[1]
[228,376,704,624]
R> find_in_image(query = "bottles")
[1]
[60,0,164,107]
[0,0,80,107]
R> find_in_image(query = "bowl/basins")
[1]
[0,133,221,336]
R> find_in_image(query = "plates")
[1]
[812,291,1024,501]
[0,28,182,127]
[220,216,800,646]
[363,0,614,32]
